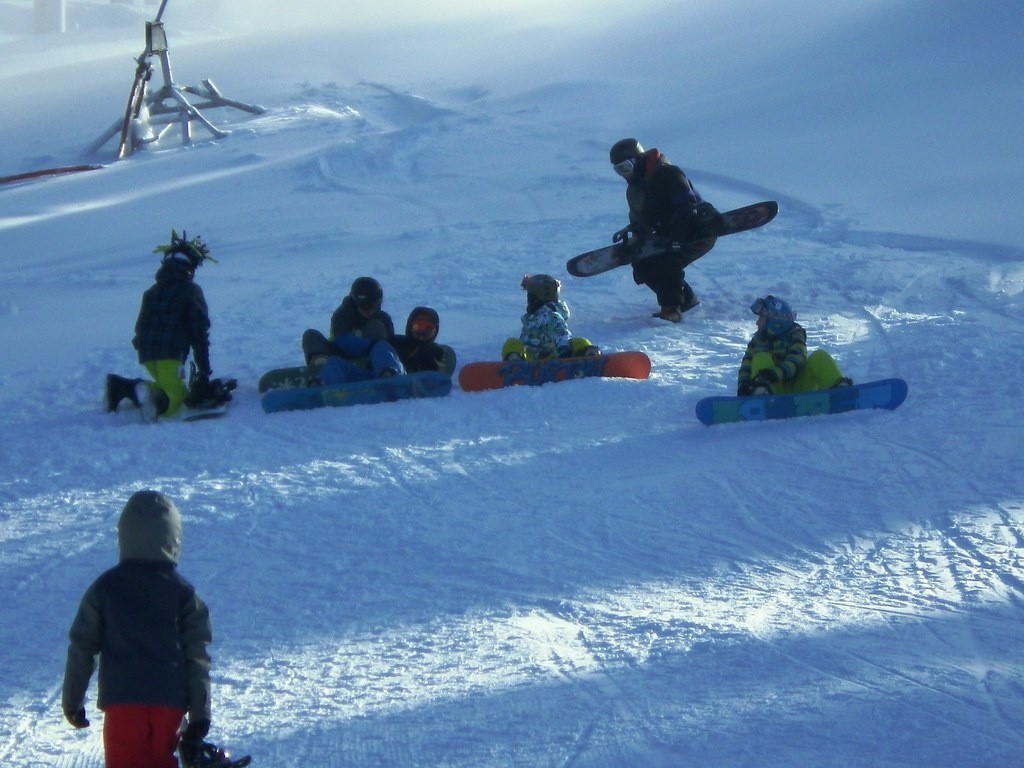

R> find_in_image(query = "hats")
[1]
[153,229,220,269]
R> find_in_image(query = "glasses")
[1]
[614,157,637,177]
[520,274,533,287]
[411,320,436,334]
[750,298,768,318]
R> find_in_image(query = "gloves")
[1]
[200,374,209,384]
[181,719,210,739]
[737,383,754,396]
[557,345,571,358]
[65,707,90,728]
[759,368,778,383]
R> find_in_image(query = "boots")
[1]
[102,373,144,414]
[136,380,169,424]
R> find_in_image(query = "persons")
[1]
[61,488,211,768]
[299,275,439,384]
[610,138,717,322]
[503,273,590,362]
[102,229,214,425]
[735,295,851,397]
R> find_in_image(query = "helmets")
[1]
[610,138,647,182]
[350,277,384,303]
[527,274,559,304]
[764,295,797,338]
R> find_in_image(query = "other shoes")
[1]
[830,376,854,389]
[505,352,526,361]
[752,382,772,395]
[178,737,252,768]
[583,346,602,356]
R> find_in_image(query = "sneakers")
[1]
[679,283,702,319]
[651,304,682,323]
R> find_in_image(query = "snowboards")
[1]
[258,345,457,394]
[457,349,652,393]
[565,200,781,278]
[694,375,910,425]
[261,369,454,416]
[181,375,235,421]
